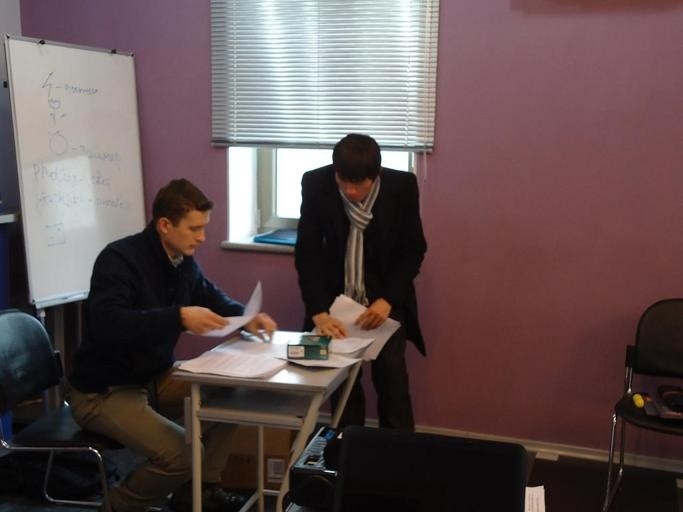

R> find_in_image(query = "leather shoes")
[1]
[201,483,247,509]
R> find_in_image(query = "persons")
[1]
[294,134,427,431]
[69,178,247,512]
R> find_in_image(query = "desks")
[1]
[169,330,363,511]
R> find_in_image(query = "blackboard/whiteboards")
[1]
[5,35,148,308]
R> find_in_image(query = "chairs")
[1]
[284,424,537,511]
[602,297,682,512]
[0,308,131,512]
